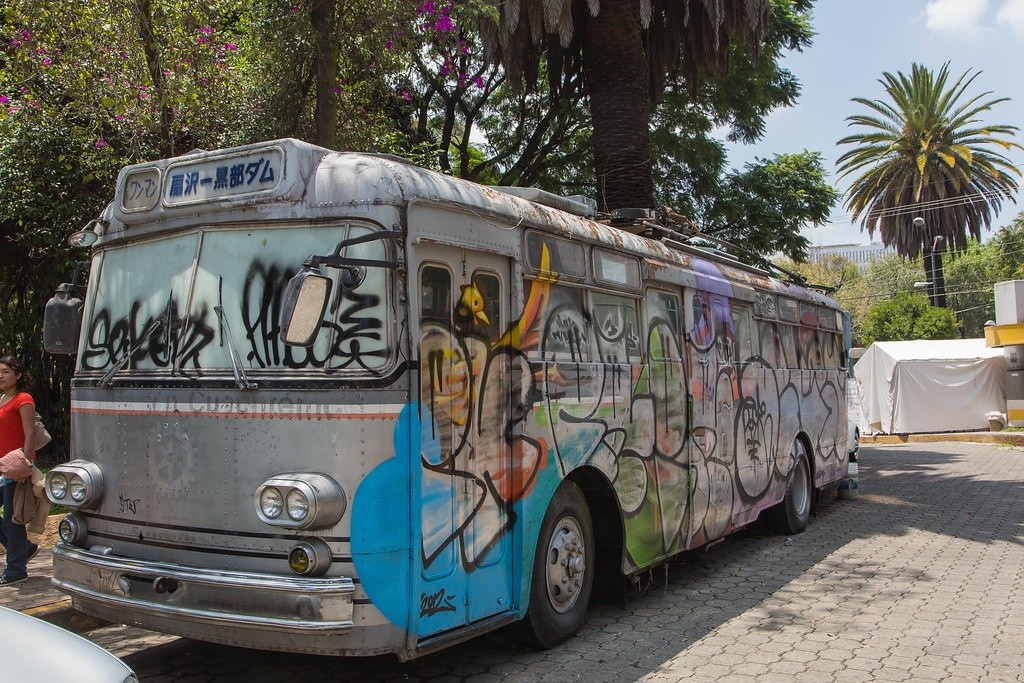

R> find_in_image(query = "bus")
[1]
[40,133,852,669]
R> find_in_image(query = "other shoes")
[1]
[0,570,29,586]
[26,543,43,564]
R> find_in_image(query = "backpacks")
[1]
[33,410,52,451]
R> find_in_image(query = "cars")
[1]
[847,416,860,461]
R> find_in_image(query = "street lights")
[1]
[912,217,944,307]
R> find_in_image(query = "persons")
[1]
[0,355,41,587]
